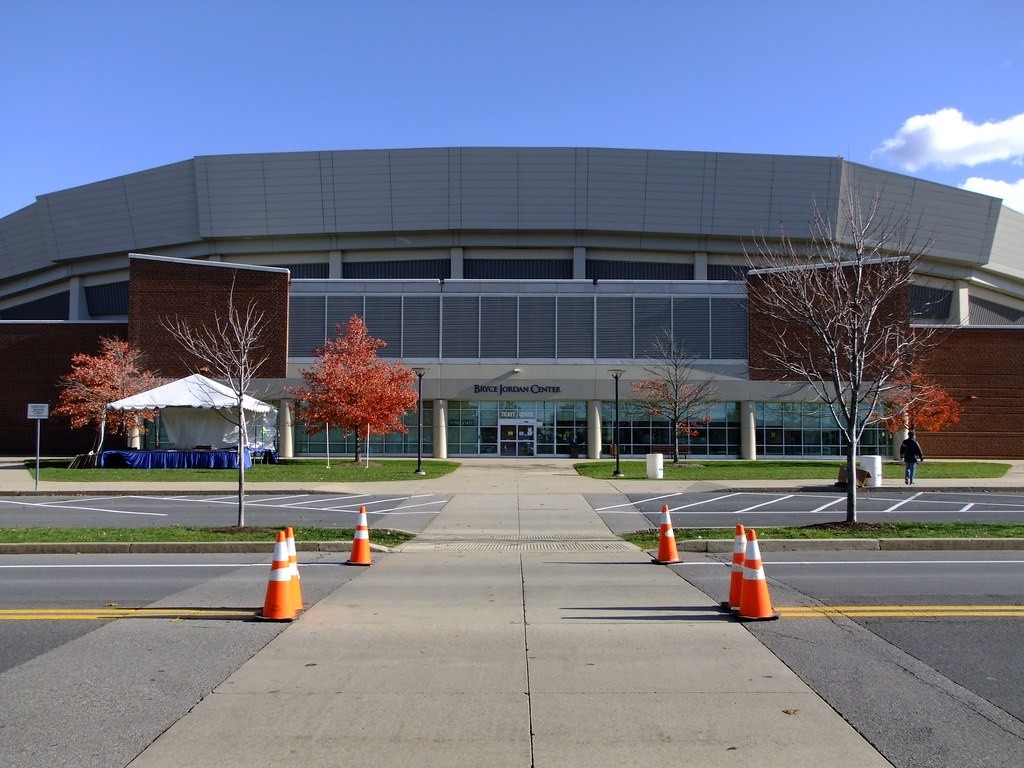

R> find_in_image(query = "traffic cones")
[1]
[345,506,372,566]
[651,505,685,564]
[286,527,303,611]
[721,525,747,609]
[731,529,780,621]
[253,531,299,620]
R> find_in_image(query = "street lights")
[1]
[607,369,628,474]
[412,368,432,472]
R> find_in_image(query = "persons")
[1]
[900,432,923,486]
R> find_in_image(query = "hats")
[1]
[908,431,915,436]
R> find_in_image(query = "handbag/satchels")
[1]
[913,455,917,463]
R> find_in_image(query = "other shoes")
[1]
[904,476,908,485]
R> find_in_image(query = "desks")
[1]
[220,446,278,466]
[100,450,252,469]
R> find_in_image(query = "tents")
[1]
[106,373,273,453]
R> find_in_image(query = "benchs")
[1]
[664,451,692,459]
